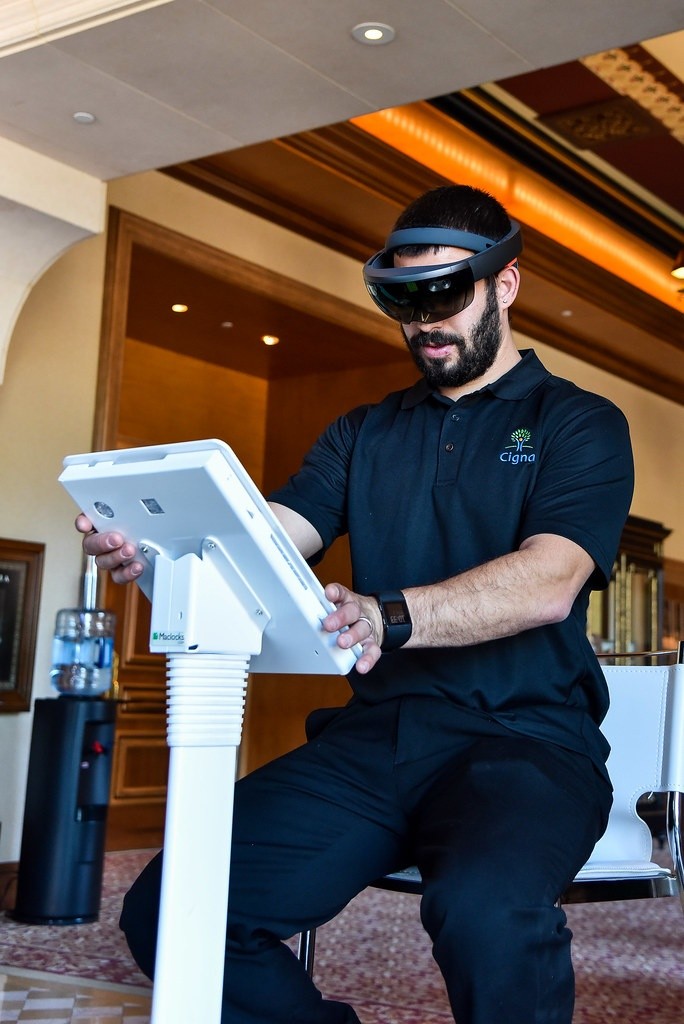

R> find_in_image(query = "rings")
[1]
[358,617,373,635]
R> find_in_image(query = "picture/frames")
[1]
[0,536,48,716]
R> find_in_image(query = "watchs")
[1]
[372,589,412,649]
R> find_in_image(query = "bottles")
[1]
[50,609,116,696]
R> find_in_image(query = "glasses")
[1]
[364,263,476,324]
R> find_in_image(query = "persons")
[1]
[74,183,635,1024]
